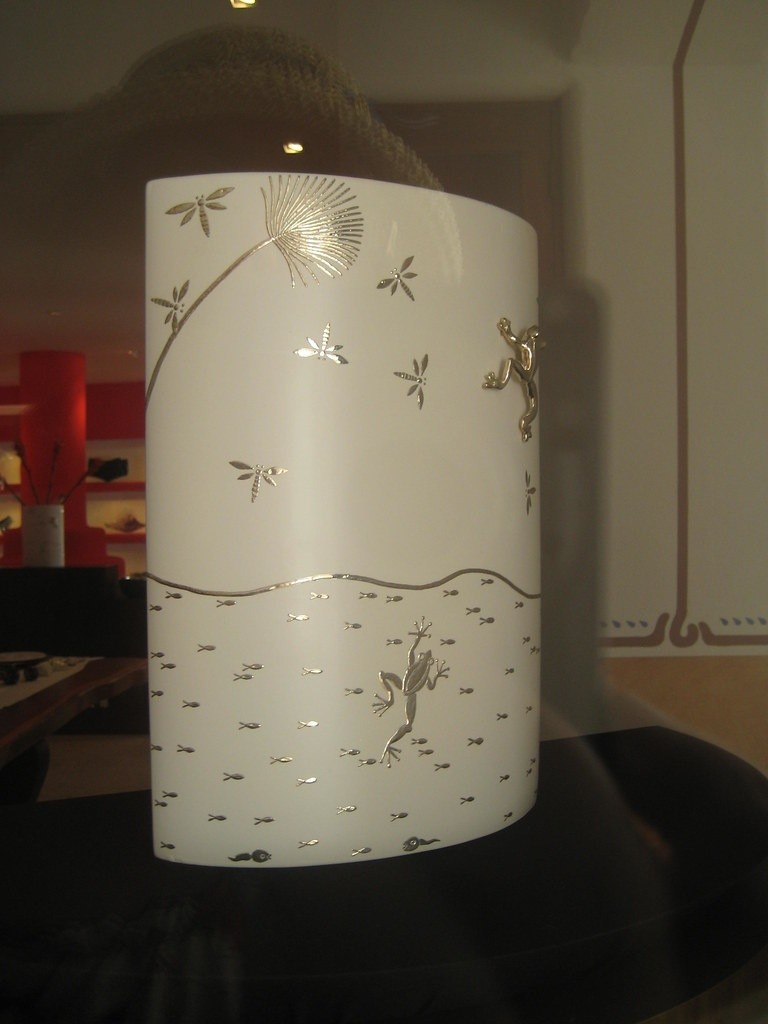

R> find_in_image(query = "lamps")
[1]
[22,503,65,568]
[143,173,544,869]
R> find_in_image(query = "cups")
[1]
[20,505,64,566]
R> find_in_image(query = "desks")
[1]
[1,652,768,1024]
